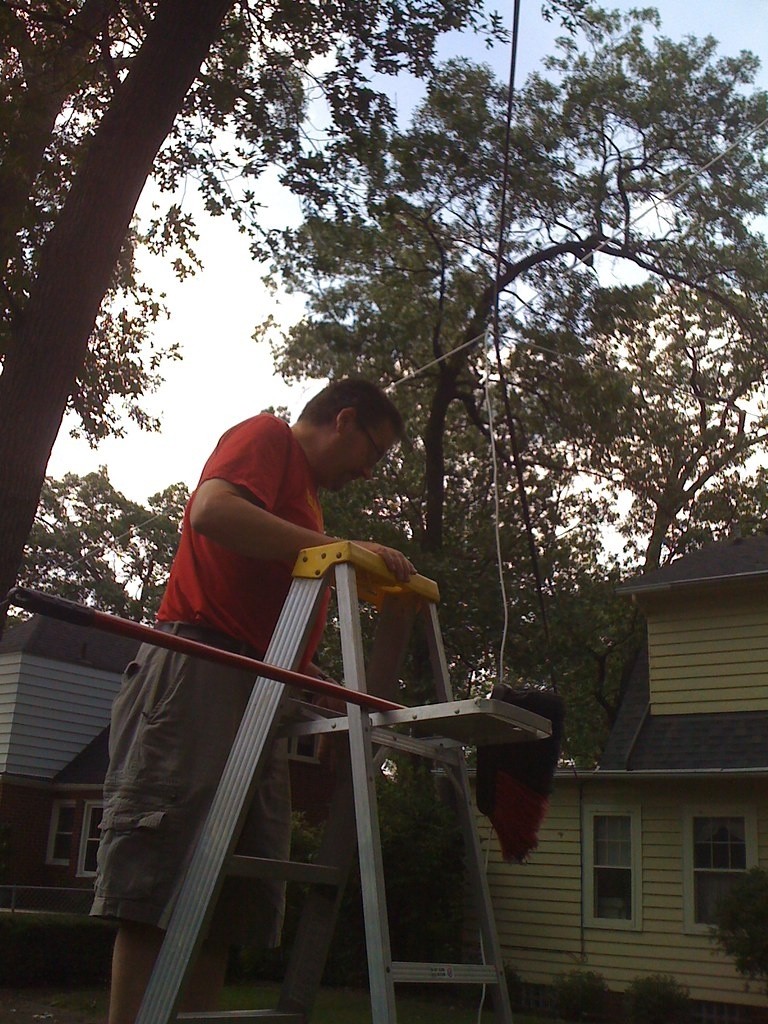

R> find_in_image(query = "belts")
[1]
[154,620,261,661]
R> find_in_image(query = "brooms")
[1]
[4,584,568,865]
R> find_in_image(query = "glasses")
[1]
[357,419,387,463]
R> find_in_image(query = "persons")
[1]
[87,377,417,1024]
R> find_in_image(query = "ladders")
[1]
[133,541,557,1024]
